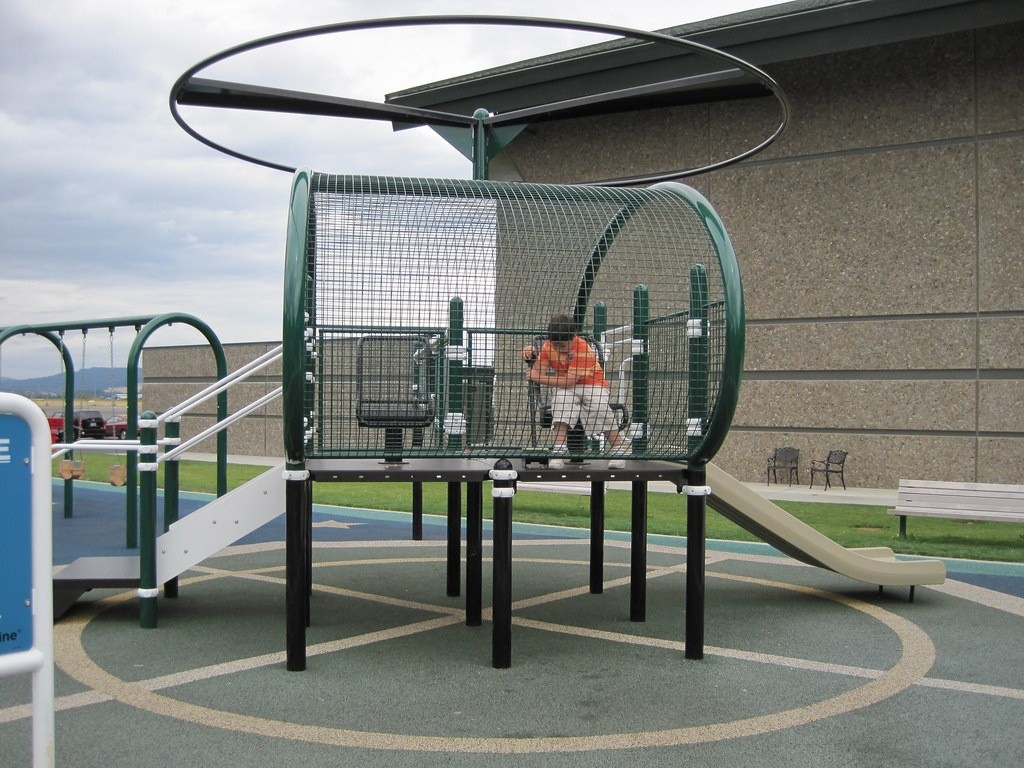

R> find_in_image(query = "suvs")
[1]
[73,409,107,440]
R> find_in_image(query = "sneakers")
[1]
[548,448,571,469]
[608,449,627,468]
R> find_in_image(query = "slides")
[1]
[670,443,948,588]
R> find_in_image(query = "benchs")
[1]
[886,479,1024,538]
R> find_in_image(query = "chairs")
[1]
[532,336,629,452]
[356,336,433,465]
[809,449,848,492]
[766,447,800,487]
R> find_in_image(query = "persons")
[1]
[522,315,627,470]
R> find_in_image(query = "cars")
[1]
[104,413,140,439]
[47,411,65,453]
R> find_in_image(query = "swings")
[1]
[109,334,129,489]
[59,336,86,481]
